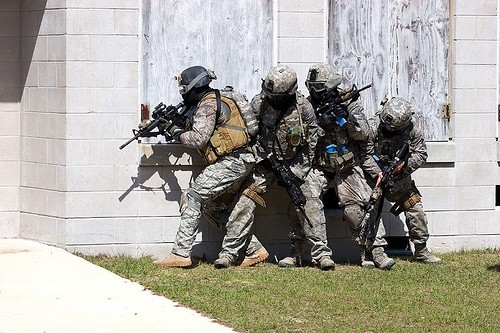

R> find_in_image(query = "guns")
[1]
[315,83,374,119]
[357,142,408,228]
[256,135,313,229]
[119,101,185,151]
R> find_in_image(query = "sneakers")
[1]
[236,246,269,266]
[214,254,236,267]
[278,257,304,267]
[361,247,397,269]
[313,256,335,270]
[412,250,442,264]
[153,253,192,267]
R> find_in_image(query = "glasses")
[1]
[307,82,326,92]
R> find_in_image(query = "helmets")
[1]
[306,63,336,81]
[381,97,413,131]
[261,65,298,94]
[178,66,212,88]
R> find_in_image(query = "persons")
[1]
[277,63,395,271]
[359,96,441,267]
[214,64,335,270]
[153,66,269,268]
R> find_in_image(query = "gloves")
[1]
[156,106,193,141]
